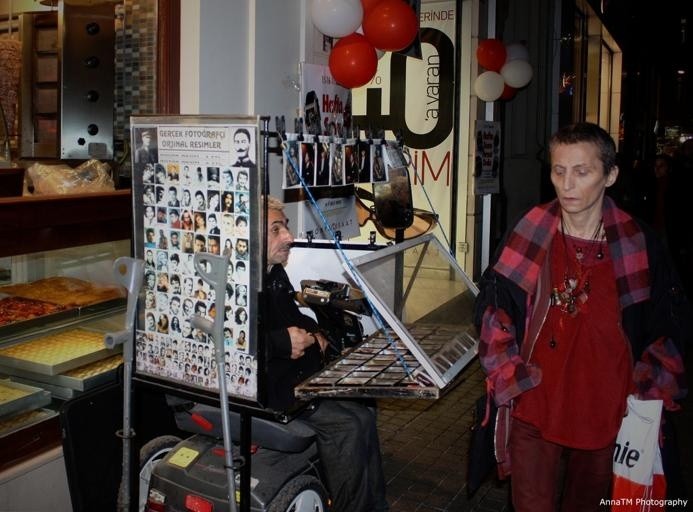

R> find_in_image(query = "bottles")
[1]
[113,133,132,190]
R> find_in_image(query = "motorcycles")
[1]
[105,251,390,512]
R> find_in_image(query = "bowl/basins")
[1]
[0,169,25,197]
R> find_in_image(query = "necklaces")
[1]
[546,209,606,351]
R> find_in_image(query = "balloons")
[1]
[361,0,417,52]
[474,71,504,102]
[476,38,507,71]
[310,0,364,39]
[506,44,535,64]
[329,33,378,88]
[501,59,534,89]
[501,84,516,101]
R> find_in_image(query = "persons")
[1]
[471,121,692,512]
[259,194,389,512]
[133,163,261,400]
[135,130,155,163]
[229,128,255,167]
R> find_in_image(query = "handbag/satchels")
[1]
[612,395,668,512]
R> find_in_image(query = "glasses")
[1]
[353,186,439,240]
